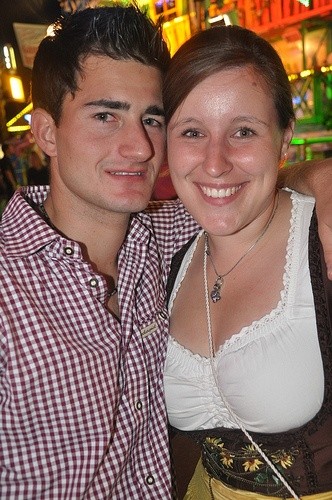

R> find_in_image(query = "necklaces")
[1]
[204,188,279,303]
[41,202,55,230]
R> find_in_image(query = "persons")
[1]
[0,0,172,500]
[163,24,332,500]
